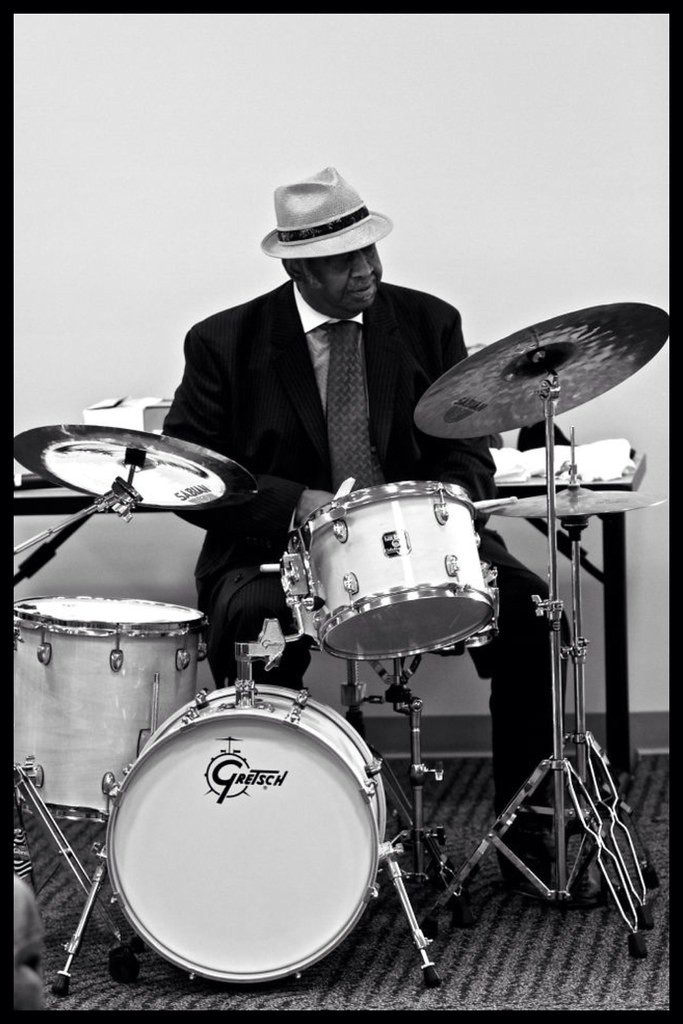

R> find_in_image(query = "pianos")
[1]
[491,445,649,492]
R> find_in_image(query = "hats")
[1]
[260,167,394,259]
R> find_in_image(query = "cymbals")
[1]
[14,423,261,512]
[411,302,669,440]
[478,482,669,518]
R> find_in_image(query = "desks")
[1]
[14,450,648,791]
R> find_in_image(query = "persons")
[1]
[160,166,609,908]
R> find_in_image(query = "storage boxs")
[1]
[83,399,172,433]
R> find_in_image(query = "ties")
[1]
[319,319,375,495]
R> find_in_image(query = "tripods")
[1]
[359,365,658,961]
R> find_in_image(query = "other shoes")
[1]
[496,830,602,910]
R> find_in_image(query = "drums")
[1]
[102,684,387,986]
[14,595,208,824]
[277,478,499,662]
